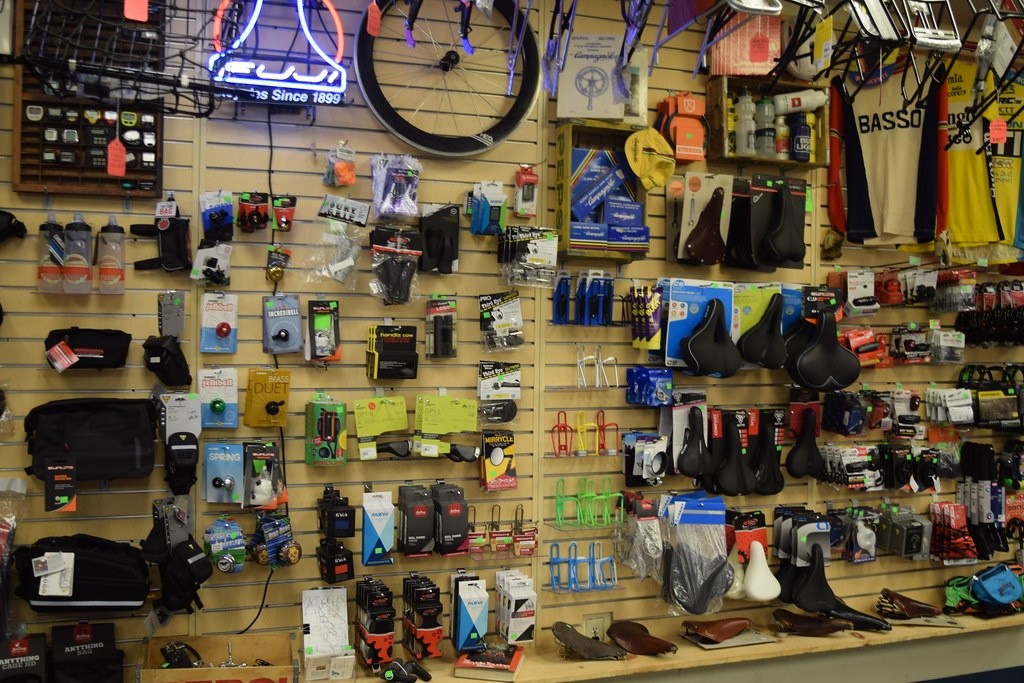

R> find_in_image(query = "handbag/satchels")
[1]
[44,327,131,372]
[944,563,1024,609]
[11,532,149,611]
[24,397,158,480]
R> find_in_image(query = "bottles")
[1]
[36,214,63,291]
[737,86,757,157]
[93,215,127,294]
[727,92,737,157]
[774,89,829,161]
[65,212,93,294]
[755,95,776,160]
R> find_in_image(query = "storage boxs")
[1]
[140,633,293,683]
[569,10,780,251]
